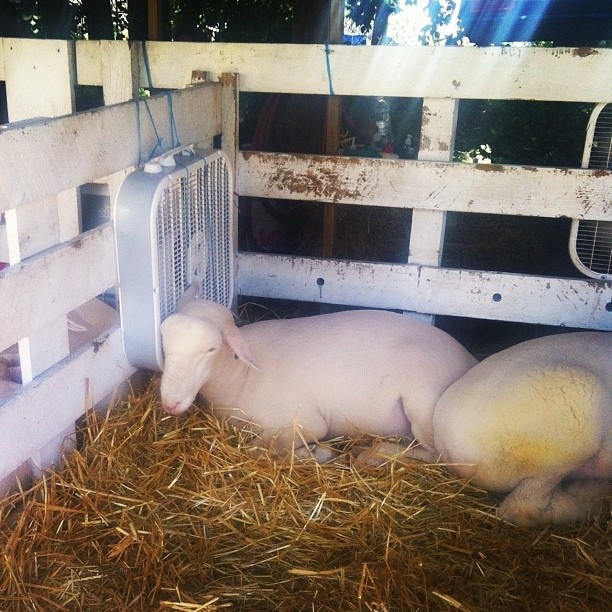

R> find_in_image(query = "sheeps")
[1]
[435,331,612,523]
[158,281,481,466]
